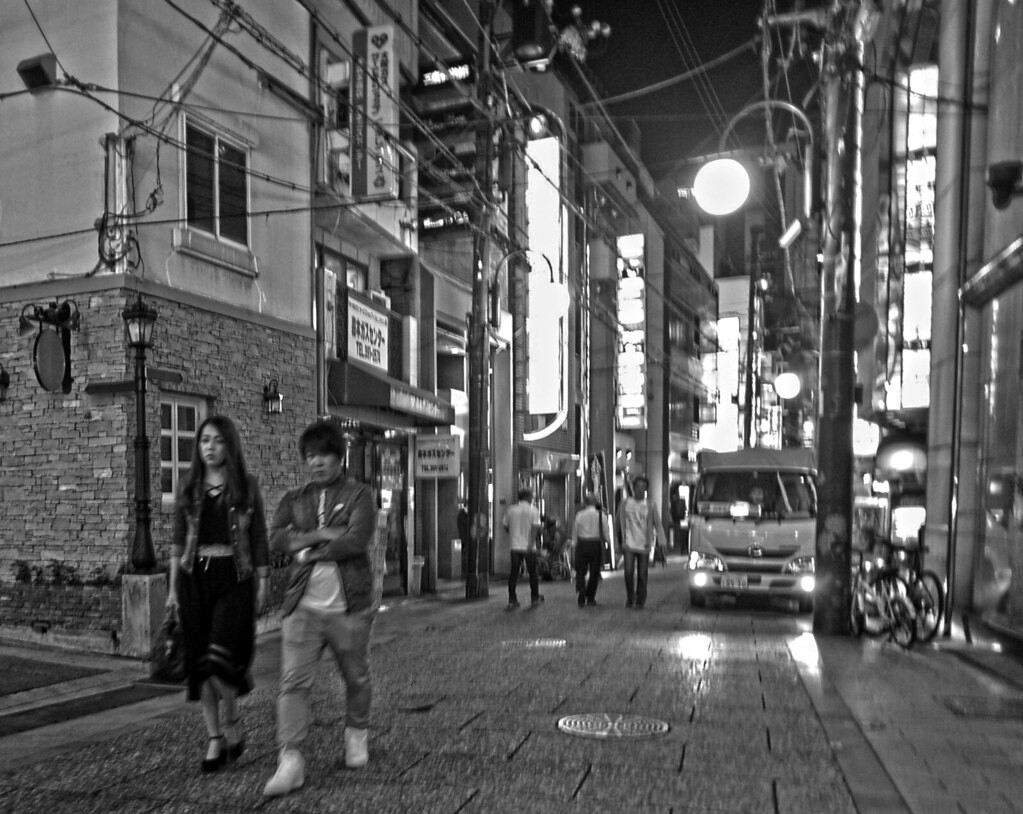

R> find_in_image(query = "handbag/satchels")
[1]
[601,541,606,566]
[150,605,185,680]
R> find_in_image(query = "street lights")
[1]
[466,247,571,600]
[744,373,801,450]
[122,292,168,660]
[693,100,855,638]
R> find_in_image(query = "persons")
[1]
[164,415,269,773]
[503,489,545,608]
[570,495,610,607]
[457,502,468,554]
[263,421,377,794]
[613,477,668,608]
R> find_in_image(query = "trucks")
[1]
[670,449,818,614]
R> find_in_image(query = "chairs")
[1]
[775,486,799,511]
[708,476,737,501]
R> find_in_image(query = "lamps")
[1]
[263,379,283,414]
[18,298,80,394]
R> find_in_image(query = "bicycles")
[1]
[849,532,917,649]
[878,536,944,643]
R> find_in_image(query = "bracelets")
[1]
[259,573,270,578]
[297,547,311,562]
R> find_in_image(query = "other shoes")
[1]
[226,715,245,761]
[531,594,544,605]
[578,588,586,607]
[508,602,520,608]
[588,599,596,606]
[636,601,644,607]
[201,733,226,773]
[626,600,633,607]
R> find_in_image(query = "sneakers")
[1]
[343,725,370,768]
[263,749,306,796]
